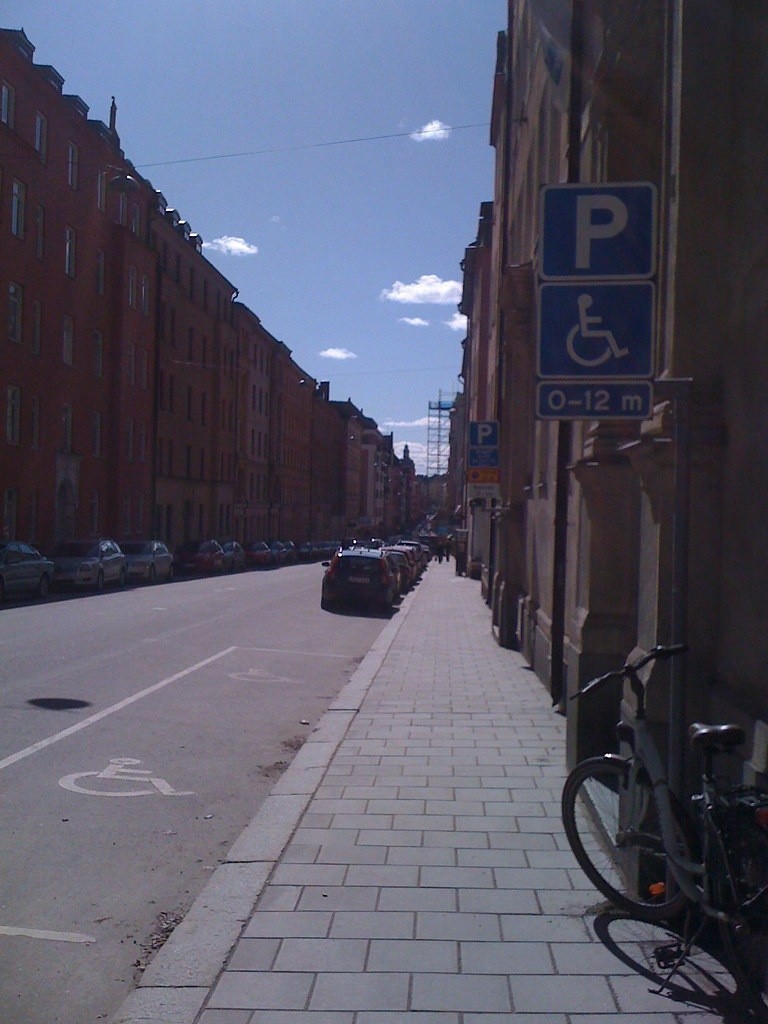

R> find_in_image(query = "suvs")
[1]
[319,546,396,612]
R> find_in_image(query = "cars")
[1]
[174,539,227,577]
[269,534,439,594]
[242,541,272,571]
[118,540,174,583]
[222,541,247,572]
[0,541,56,603]
[51,536,128,593]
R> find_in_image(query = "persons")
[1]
[435,538,452,563]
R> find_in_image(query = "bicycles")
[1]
[560,639,768,1024]
